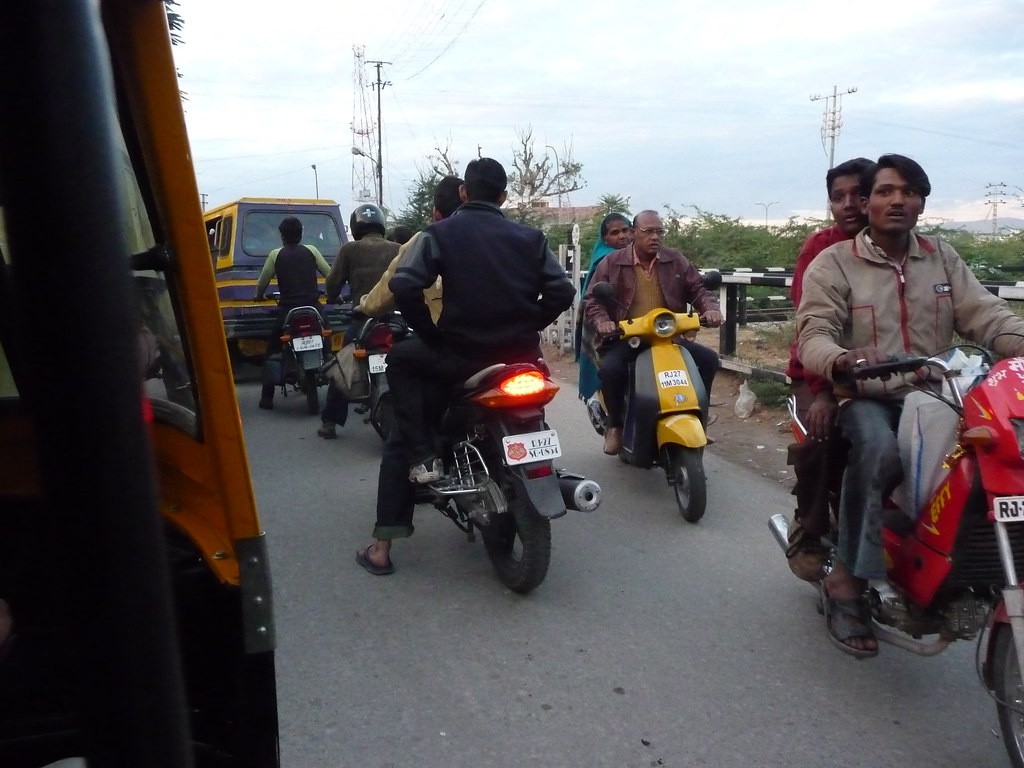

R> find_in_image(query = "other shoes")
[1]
[259,399,273,409]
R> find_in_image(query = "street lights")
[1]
[311,165,318,200]
[350,145,383,209]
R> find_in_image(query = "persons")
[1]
[574,210,722,458]
[386,225,412,245]
[317,207,402,439]
[255,218,331,409]
[783,153,1023,660]
[383,159,575,485]
[351,175,465,576]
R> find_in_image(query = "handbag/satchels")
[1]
[319,340,366,398]
[888,348,988,520]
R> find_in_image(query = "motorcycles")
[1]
[577,268,727,524]
[344,306,603,595]
[251,277,343,417]
[322,285,414,439]
[767,331,1024,768]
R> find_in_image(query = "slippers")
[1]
[819,577,878,657]
[356,543,394,575]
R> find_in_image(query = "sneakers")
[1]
[786,518,824,582]
[317,422,336,439]
[408,458,444,484]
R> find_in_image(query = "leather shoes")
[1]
[604,426,622,455]
[706,436,716,444]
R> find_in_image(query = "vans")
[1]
[201,197,356,374]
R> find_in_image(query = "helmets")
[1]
[350,204,385,239]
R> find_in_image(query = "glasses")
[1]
[634,227,666,236]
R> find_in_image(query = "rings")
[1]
[856,358,867,364]
